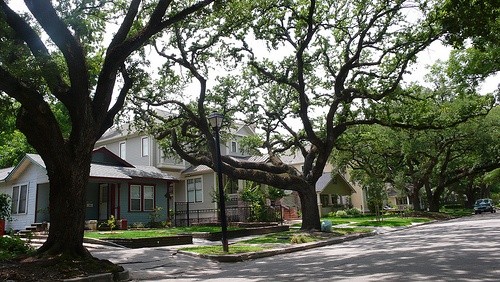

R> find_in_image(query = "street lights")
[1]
[206,109,229,253]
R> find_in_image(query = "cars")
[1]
[473,198,496,215]
